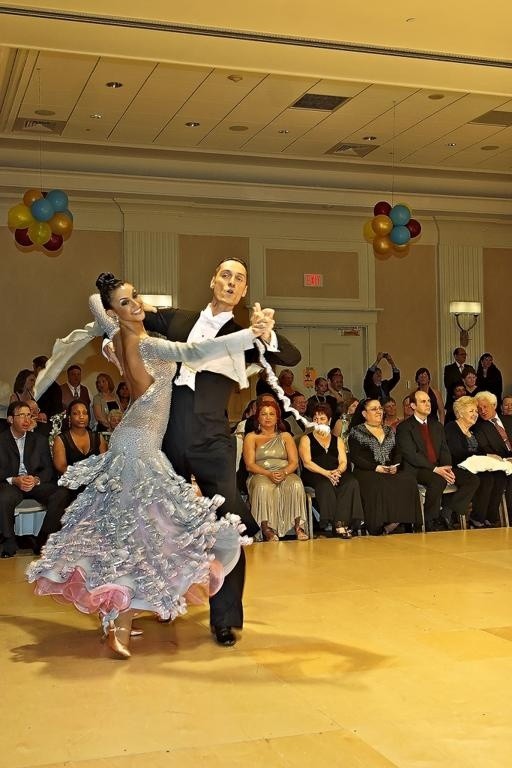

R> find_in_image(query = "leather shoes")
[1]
[216,626,235,645]
[336,526,353,538]
[440,511,455,530]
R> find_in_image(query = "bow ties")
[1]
[200,311,224,330]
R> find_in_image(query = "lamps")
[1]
[137,293,172,318]
[449,300,483,347]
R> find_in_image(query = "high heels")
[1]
[468,517,494,529]
[99,611,144,657]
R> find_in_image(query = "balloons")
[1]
[8,188,74,257]
[363,200,423,260]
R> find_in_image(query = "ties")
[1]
[74,387,78,398]
[491,417,511,450]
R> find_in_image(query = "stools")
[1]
[491,482,511,526]
[296,486,317,539]
[420,482,472,538]
[10,500,49,521]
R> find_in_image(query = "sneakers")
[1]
[2,550,16,558]
[264,528,279,541]
[295,527,309,540]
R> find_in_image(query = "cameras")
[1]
[381,352,388,358]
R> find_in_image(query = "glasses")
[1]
[13,413,32,418]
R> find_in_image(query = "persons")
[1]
[78,270,275,661]
[101,254,302,647]
